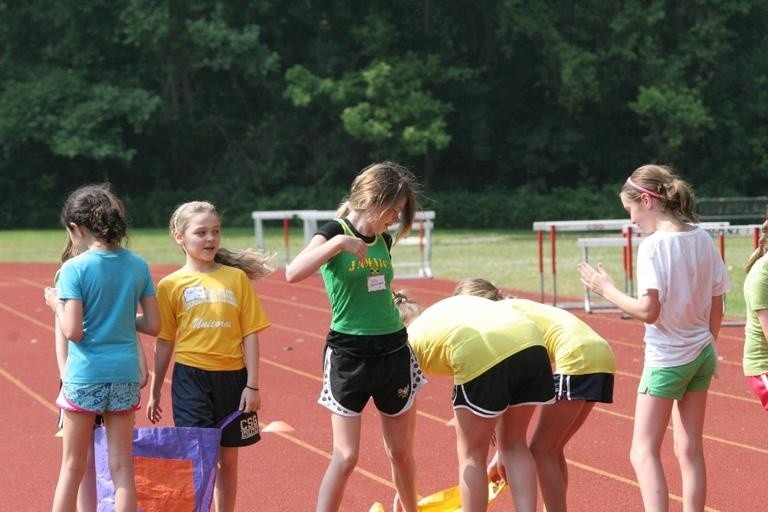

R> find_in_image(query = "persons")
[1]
[392,287,557,511]
[283,161,428,511]
[742,219,768,412]
[450,278,617,511]
[146,201,273,510]
[44,184,160,512]
[577,165,733,512]
[53,237,150,512]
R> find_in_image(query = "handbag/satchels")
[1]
[92,409,243,512]
[369,479,506,512]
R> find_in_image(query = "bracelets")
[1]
[245,384,260,391]
